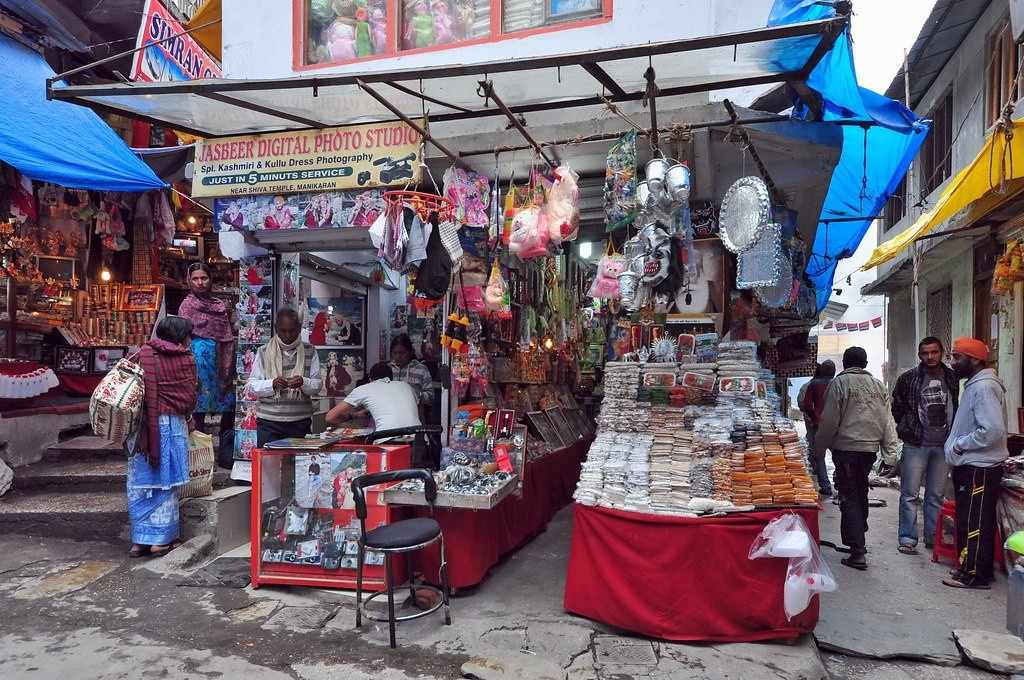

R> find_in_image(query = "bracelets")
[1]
[395,452,511,496]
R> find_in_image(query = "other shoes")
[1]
[832,498,842,505]
[819,487,832,495]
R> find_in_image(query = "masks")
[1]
[953,357,973,378]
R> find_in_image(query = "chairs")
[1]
[351,468,451,648]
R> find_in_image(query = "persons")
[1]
[325,362,436,467]
[240,286,269,314]
[347,192,379,227]
[240,257,267,285]
[222,201,250,231]
[304,194,334,228]
[354,2,453,57]
[808,346,897,570]
[245,320,262,343]
[179,263,236,438]
[310,311,361,346]
[242,349,255,372]
[240,377,256,459]
[247,306,323,449]
[307,456,354,510]
[797,359,839,495]
[891,337,959,554]
[125,317,197,558]
[941,337,1010,590]
[354,332,435,429]
[324,351,352,397]
[262,196,291,229]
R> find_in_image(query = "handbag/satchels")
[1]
[484,254,514,319]
[87,350,146,445]
[586,234,627,301]
[507,195,551,259]
[178,428,216,499]
[546,162,581,243]
[218,428,234,470]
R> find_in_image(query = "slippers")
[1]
[949,568,972,576]
[896,542,918,555]
[841,558,869,569]
[151,542,179,557]
[128,544,151,557]
[942,577,991,589]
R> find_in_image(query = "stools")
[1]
[932,501,1002,570]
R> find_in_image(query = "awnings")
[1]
[861,118,1024,273]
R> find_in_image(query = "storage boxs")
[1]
[384,474,519,510]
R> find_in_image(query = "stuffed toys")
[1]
[592,255,624,300]
[327,17,356,61]
[93,212,111,234]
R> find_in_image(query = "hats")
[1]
[326,352,338,361]
[346,467,354,475]
[330,1,359,16]
[950,336,988,362]
[355,191,374,203]
[815,360,836,379]
[250,320,256,326]
[325,305,334,315]
[844,347,868,368]
[273,196,285,204]
